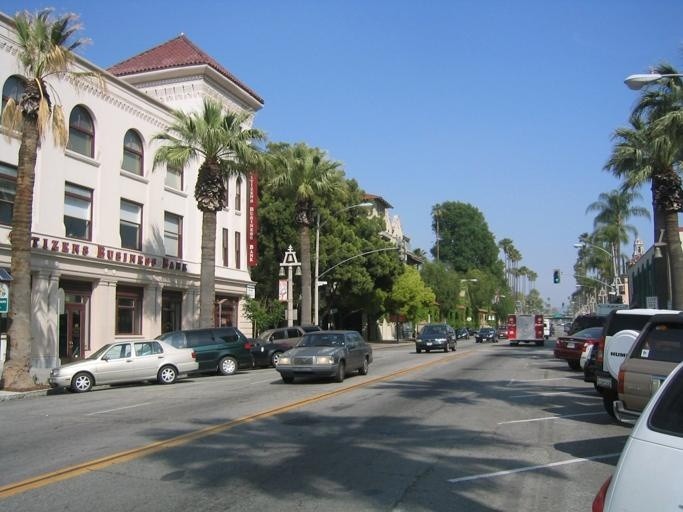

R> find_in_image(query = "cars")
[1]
[456,329,469,340]
[475,328,508,343]
[554,309,683,512]
[416,324,456,353]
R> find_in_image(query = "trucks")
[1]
[508,314,550,346]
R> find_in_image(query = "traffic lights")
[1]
[554,270,560,283]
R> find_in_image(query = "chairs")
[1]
[127,352,131,357]
[152,349,162,354]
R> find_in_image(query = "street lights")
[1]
[574,244,620,305]
[315,203,373,325]
[654,227,672,309]
[624,74,683,90]
[279,244,302,327]
